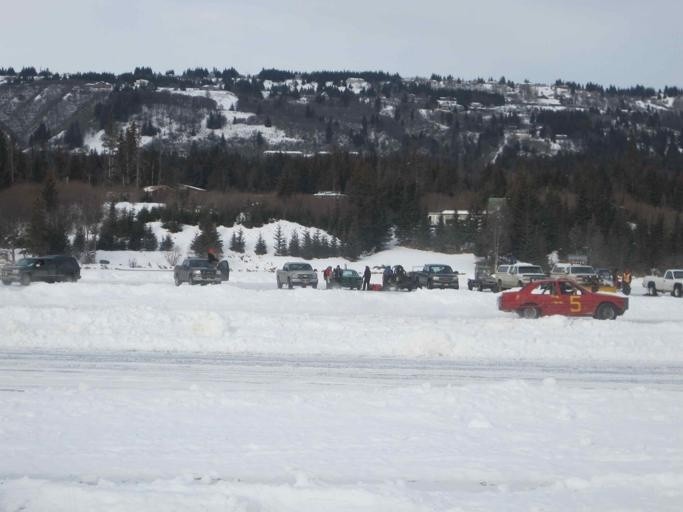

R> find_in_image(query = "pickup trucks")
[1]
[0,255,82,285]
[174,257,230,285]
[409,264,459,290]
[641,269,683,298]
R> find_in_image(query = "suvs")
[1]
[276,262,319,290]
[492,263,547,289]
[549,262,598,285]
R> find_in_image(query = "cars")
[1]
[594,267,617,288]
[325,269,363,290]
[499,279,629,319]
[467,272,497,290]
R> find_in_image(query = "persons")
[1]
[323,266,333,289]
[382,265,392,289]
[360,266,371,290]
[621,268,632,296]
[333,265,344,289]
